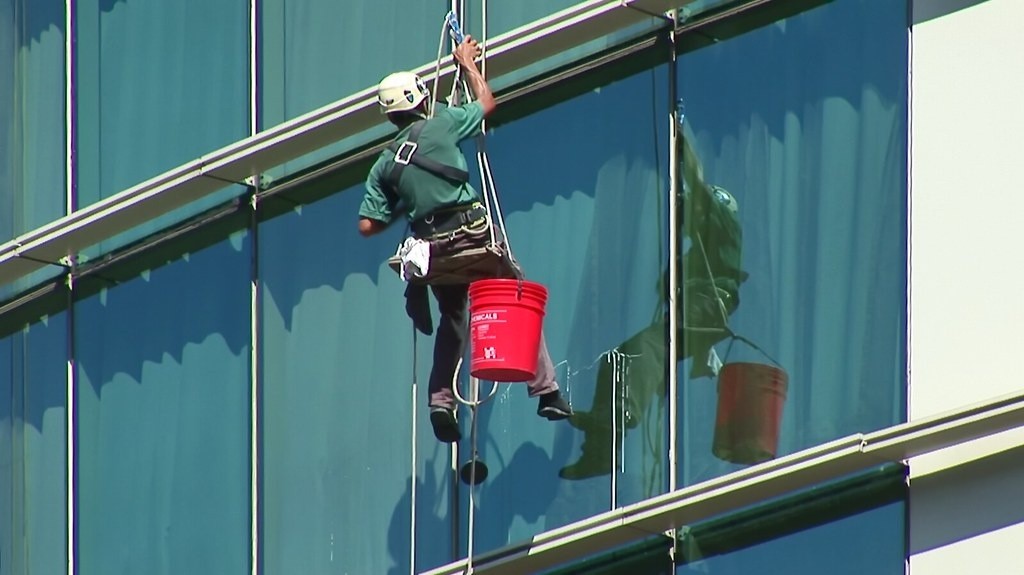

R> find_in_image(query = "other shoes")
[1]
[430,405,463,443]
[558,455,613,480]
[538,390,572,421]
[569,411,626,443]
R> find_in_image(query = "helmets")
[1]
[710,184,738,213]
[378,71,430,113]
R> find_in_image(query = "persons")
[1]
[358,35,574,442]
[557,115,743,481]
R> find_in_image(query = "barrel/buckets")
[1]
[712,336,789,467]
[469,265,548,383]
